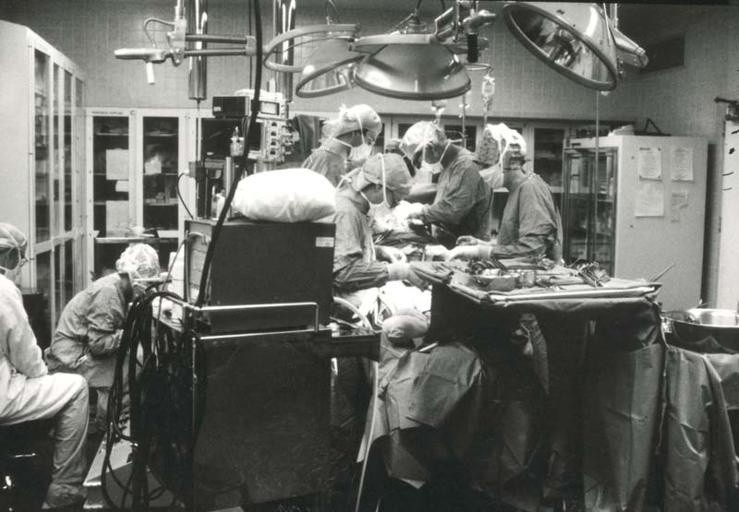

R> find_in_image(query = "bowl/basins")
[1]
[662,308,739,353]
[472,270,521,292]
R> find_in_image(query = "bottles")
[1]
[230,125,244,157]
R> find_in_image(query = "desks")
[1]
[411,254,663,512]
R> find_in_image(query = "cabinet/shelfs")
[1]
[560,128,711,319]
[77,101,638,295]
[0,18,90,364]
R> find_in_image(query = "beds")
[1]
[325,250,484,495]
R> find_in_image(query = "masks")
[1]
[348,128,373,163]
[417,142,450,175]
[478,154,505,189]
[0,248,26,281]
[359,179,395,225]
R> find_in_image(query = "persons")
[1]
[50,242,163,434]
[0,223,90,510]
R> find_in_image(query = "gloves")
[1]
[444,244,494,263]
[387,264,431,292]
[374,245,408,264]
